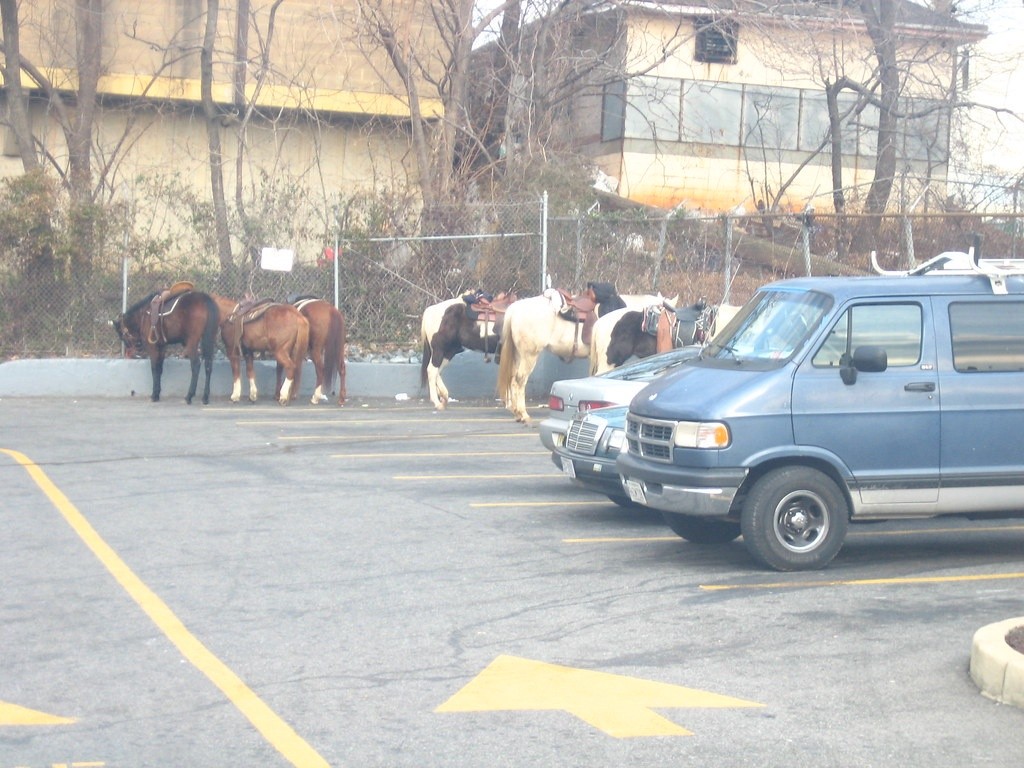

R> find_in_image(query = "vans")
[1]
[616,231,1024,572]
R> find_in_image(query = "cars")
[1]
[559,404,632,496]
[537,345,708,455]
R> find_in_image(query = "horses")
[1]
[112,281,221,406]
[417,288,531,411]
[209,293,310,407]
[290,295,347,409]
[498,281,679,429]
[587,300,743,377]
